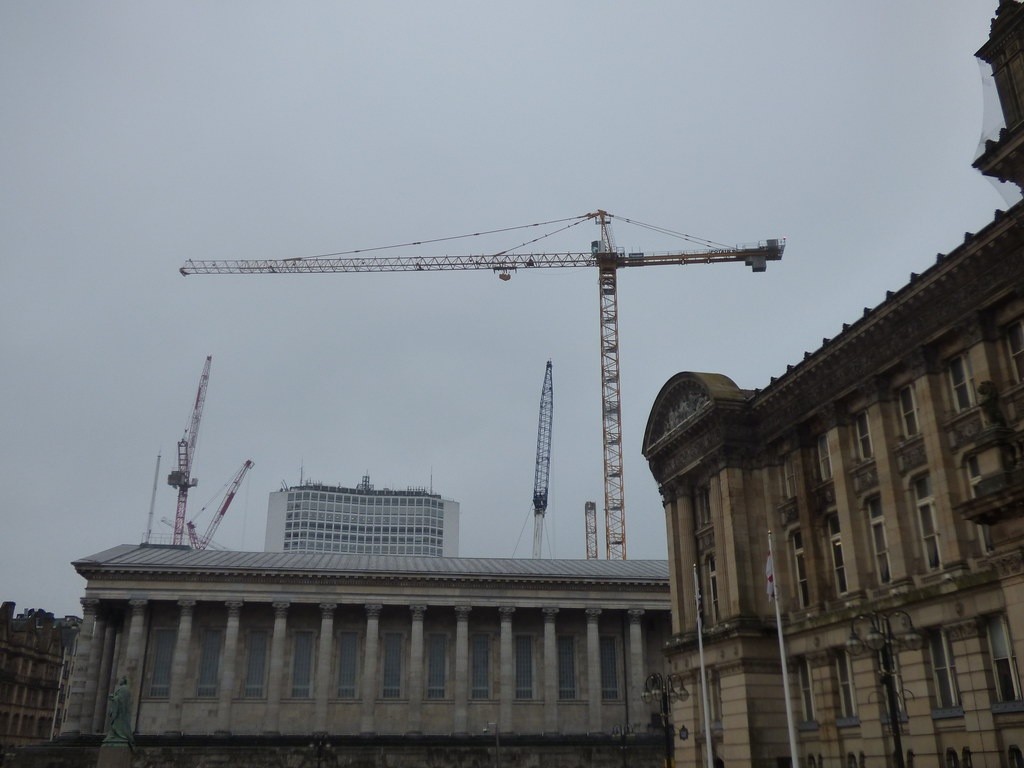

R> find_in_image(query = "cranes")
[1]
[180,207,787,560]
[165,353,253,550]
[529,359,553,560]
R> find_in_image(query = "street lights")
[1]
[611,723,637,768]
[308,732,333,768]
[640,673,672,768]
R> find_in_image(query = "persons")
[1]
[664,391,708,434]
[102,678,133,746]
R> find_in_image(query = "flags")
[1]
[693,563,705,628]
[765,530,779,603]
